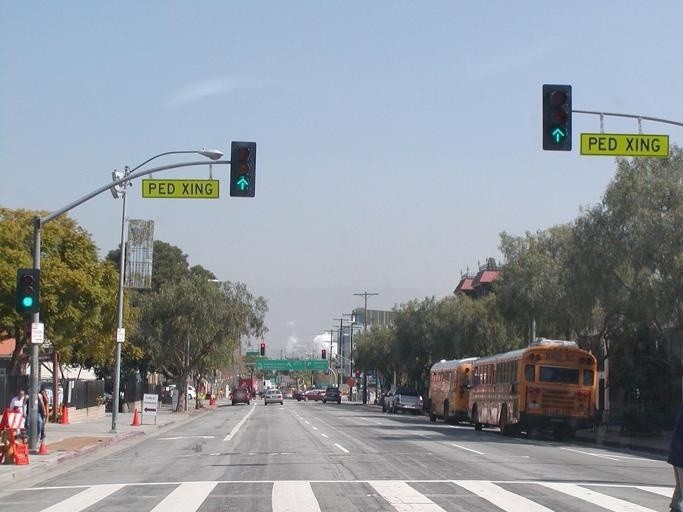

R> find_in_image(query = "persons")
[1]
[665,407,682,511]
[8,390,25,411]
[20,389,30,442]
[34,387,49,442]
[35,391,46,442]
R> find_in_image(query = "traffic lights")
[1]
[228,140,258,199]
[541,84,573,153]
[15,269,41,316]
[260,343,266,356]
[321,349,327,360]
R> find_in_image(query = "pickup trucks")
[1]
[389,386,425,414]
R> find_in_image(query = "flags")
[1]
[121,218,154,291]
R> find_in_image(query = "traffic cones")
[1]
[59,405,70,425]
[130,408,141,427]
[37,434,50,456]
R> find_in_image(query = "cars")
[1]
[231,383,342,405]
[382,387,396,412]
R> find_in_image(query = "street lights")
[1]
[108,147,224,431]
[184,276,223,411]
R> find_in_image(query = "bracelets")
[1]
[42,414,46,418]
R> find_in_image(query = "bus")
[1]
[425,355,481,426]
[463,339,599,438]
